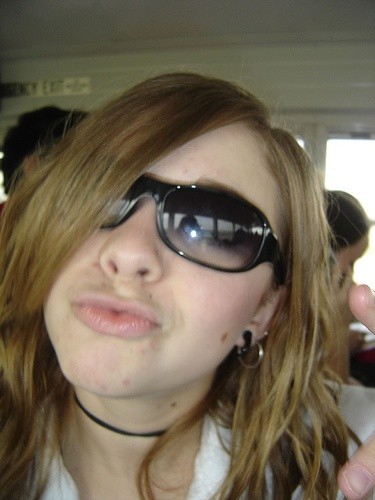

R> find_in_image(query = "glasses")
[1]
[100,175,287,286]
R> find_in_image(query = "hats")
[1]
[323,191,375,251]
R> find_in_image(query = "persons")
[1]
[0,68,375,500]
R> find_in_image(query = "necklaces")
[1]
[71,383,167,440]
